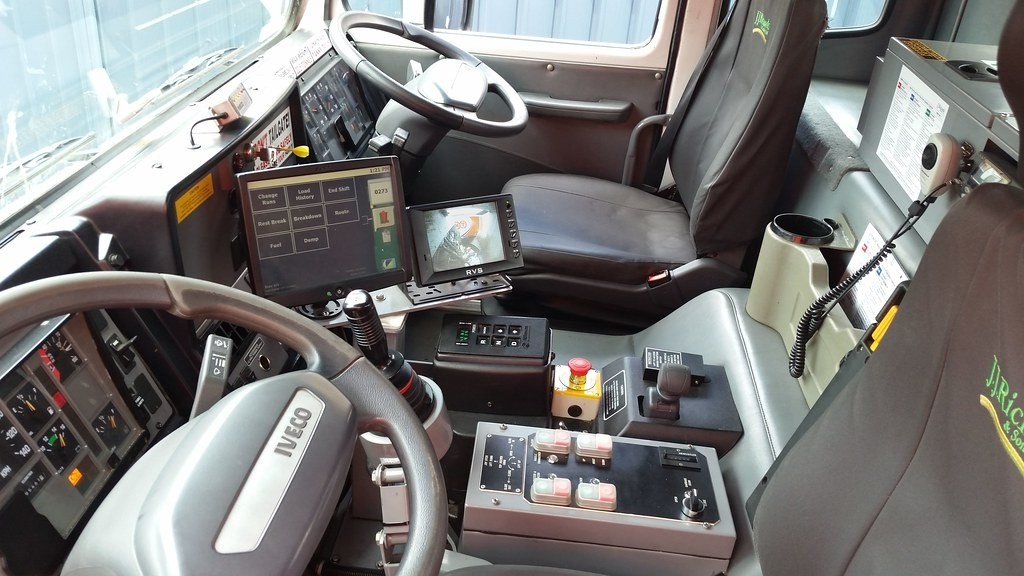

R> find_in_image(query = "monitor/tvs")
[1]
[234,156,414,308]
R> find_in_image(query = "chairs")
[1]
[438,1,1024,576]
[499,0,827,334]
